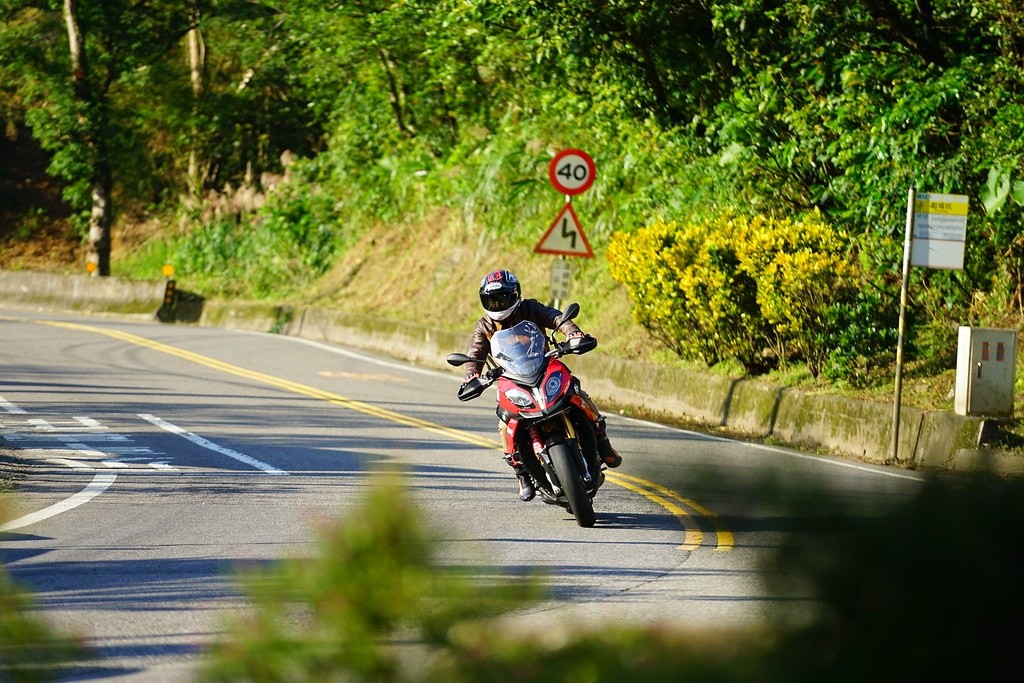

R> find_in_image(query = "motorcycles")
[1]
[442,302,614,529]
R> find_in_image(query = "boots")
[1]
[595,427,622,468]
[513,463,536,501]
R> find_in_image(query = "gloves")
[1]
[462,373,480,388]
[564,332,585,352]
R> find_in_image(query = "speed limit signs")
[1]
[545,147,597,195]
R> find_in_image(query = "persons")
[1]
[463,269,622,501]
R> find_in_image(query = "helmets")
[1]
[479,270,521,321]
